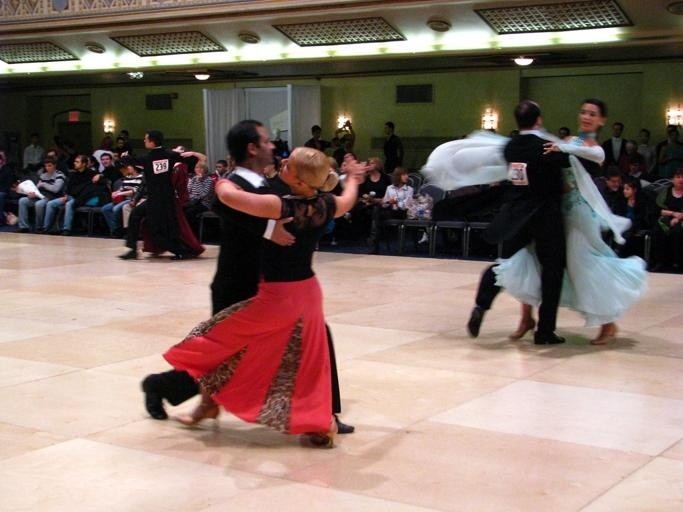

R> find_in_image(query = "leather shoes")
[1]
[534,330,565,345]
[469,305,484,338]
[141,370,168,421]
[119,250,136,260]
[292,413,357,449]
[175,403,220,427]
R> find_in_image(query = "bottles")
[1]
[383,196,409,210]
[417,202,432,221]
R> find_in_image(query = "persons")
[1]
[142,120,365,433]
[161,147,362,450]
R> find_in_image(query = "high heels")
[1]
[510,317,536,339]
[590,322,617,345]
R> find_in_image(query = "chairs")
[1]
[183,165,227,245]
[587,166,682,275]
[316,165,519,262]
[0,165,142,240]
